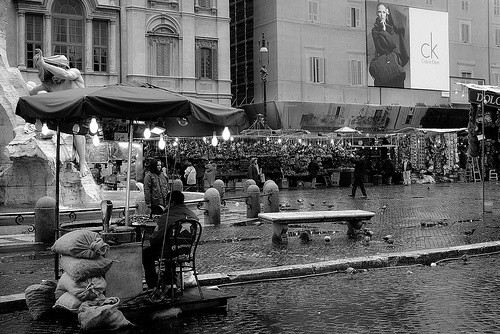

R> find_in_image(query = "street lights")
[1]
[260,29,268,129]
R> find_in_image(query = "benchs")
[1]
[258,210,376,245]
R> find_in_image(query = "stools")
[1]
[489,170,498,180]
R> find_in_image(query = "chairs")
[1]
[148,219,204,300]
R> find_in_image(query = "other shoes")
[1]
[140,287,160,298]
[160,284,178,297]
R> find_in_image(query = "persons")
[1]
[142,189,199,292]
[308,159,320,188]
[144,159,168,214]
[183,158,216,191]
[349,155,367,198]
[369,3,409,88]
[402,156,412,185]
[31,48,89,178]
[249,158,263,185]
